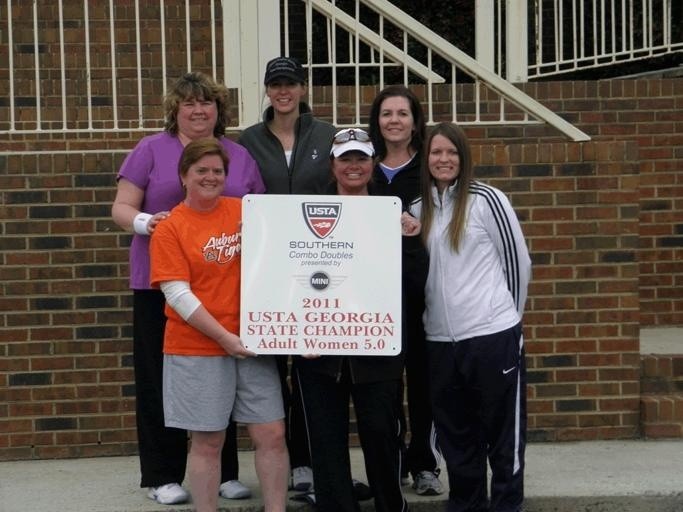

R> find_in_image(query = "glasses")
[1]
[331,129,374,147]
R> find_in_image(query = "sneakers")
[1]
[146,481,189,505]
[219,478,252,500]
[411,469,445,495]
[401,471,411,485]
[291,466,316,491]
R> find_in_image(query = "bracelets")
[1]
[132,212,154,237]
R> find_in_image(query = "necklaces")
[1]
[384,149,415,164]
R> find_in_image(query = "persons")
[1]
[111,68,266,507]
[293,127,429,511]
[409,121,534,511]
[239,56,339,490]
[147,134,291,511]
[368,84,449,495]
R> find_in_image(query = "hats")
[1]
[263,56,307,86]
[329,127,376,160]
